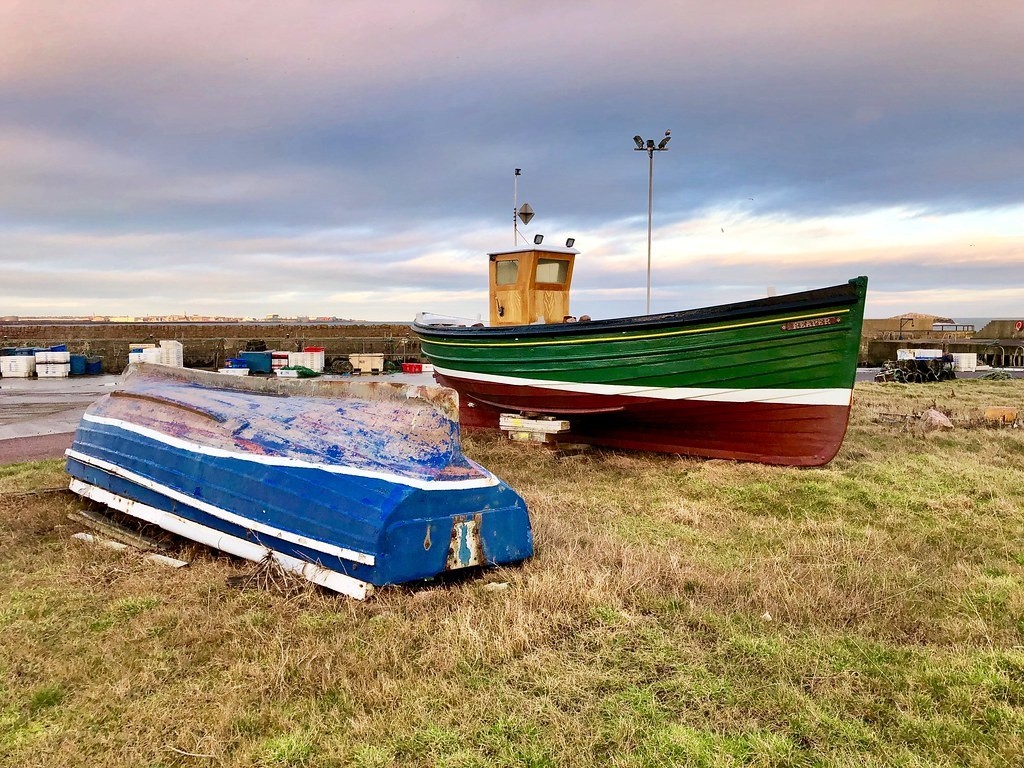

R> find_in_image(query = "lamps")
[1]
[566,237,575,247]
[534,234,544,244]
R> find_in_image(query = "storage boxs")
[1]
[0,345,101,379]
[218,346,325,378]
[402,362,433,372]
[897,348,977,372]
[350,351,384,373]
[127,339,183,368]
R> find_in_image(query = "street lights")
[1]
[632,128,671,314]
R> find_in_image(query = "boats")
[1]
[64,362,533,601]
[411,168,868,468]
